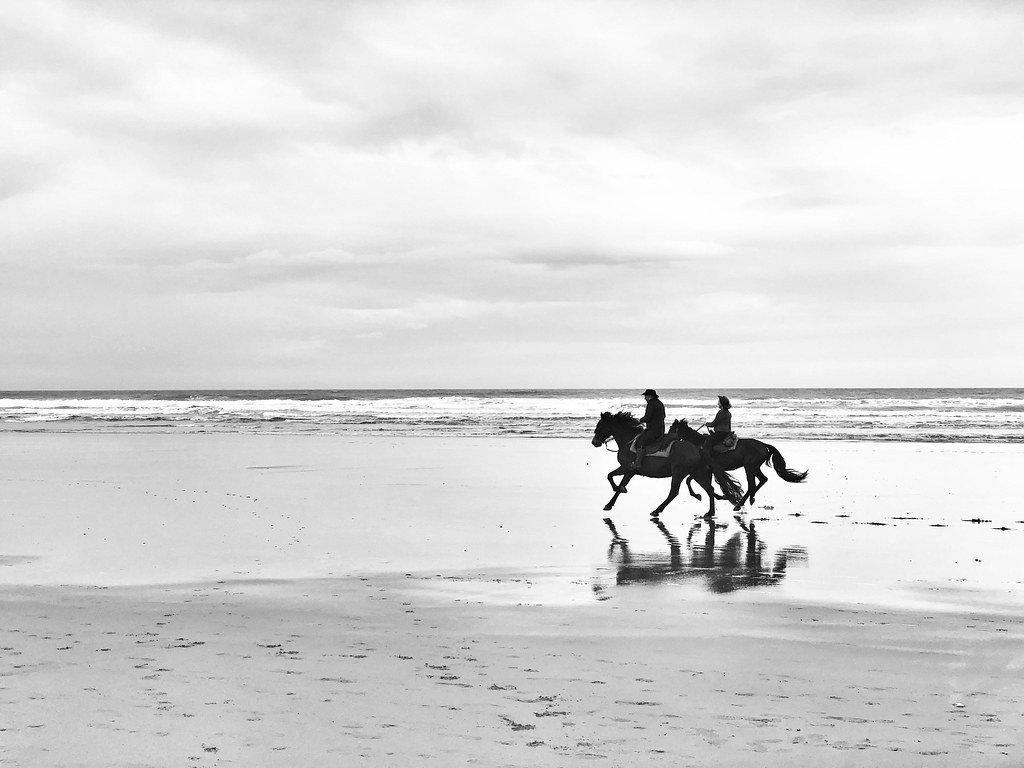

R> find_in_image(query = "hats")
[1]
[718,396,731,409]
[642,389,659,397]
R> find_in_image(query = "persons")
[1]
[703,396,731,446]
[628,390,665,470]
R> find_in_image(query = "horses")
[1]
[592,409,744,518]
[668,418,809,511]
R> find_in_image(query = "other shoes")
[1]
[703,465,710,474]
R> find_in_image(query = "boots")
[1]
[627,448,645,471]
